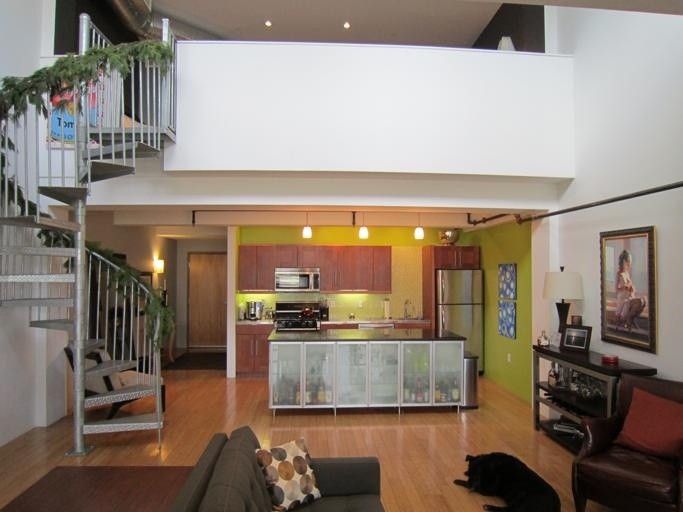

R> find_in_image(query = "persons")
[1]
[615,250,646,328]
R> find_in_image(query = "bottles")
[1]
[537,330,550,347]
[273,376,332,404]
[265,306,273,319]
[320,298,330,321]
[405,374,460,402]
[349,312,356,321]
[238,303,245,320]
[548,363,596,402]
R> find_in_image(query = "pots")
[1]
[245,300,264,321]
[436,229,460,246]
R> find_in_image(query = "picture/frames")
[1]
[559,323,593,354]
[134,272,153,319]
[600,226,660,355]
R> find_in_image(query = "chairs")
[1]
[573,371,682,511]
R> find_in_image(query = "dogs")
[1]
[453,451,561,512]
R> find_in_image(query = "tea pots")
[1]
[302,306,314,318]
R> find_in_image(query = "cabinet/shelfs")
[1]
[238,245,298,290]
[533,344,658,453]
[400,340,463,414]
[372,246,392,293]
[298,246,372,293]
[335,342,400,417]
[269,343,335,417]
[236,334,269,375]
[421,245,480,269]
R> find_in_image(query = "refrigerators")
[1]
[436,269,484,374]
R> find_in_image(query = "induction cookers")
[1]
[273,313,321,328]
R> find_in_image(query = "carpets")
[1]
[0,466,192,512]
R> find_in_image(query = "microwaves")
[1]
[274,267,320,293]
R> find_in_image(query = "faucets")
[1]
[404,299,414,320]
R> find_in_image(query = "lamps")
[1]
[155,259,164,275]
[541,265,583,334]
[359,212,368,239]
[414,212,425,240]
[302,213,312,239]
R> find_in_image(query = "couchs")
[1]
[168,426,387,512]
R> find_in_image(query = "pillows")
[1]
[254,438,323,511]
